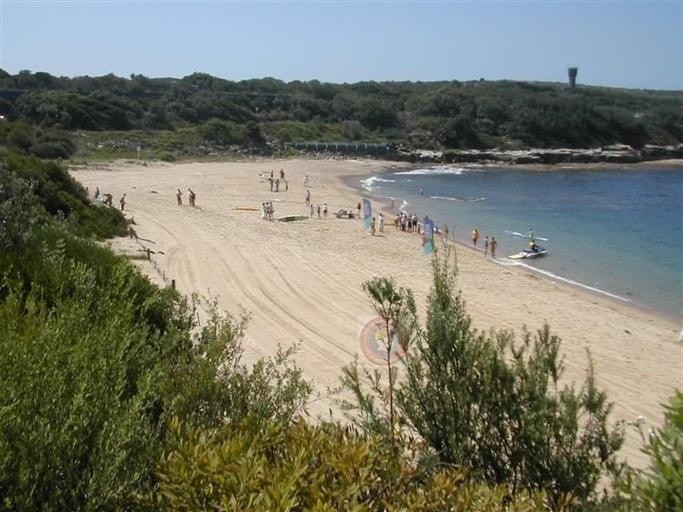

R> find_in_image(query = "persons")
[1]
[378,213,384,232]
[95,186,99,198]
[310,203,327,218]
[262,202,274,220]
[394,213,418,232]
[305,190,310,205]
[120,194,126,210]
[527,228,533,238]
[104,193,112,206]
[303,175,308,186]
[188,189,195,206]
[269,168,288,192]
[176,189,183,204]
[471,226,497,258]
[356,203,361,219]
[369,217,376,235]
[529,240,538,252]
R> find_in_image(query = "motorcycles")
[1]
[522,246,546,258]
